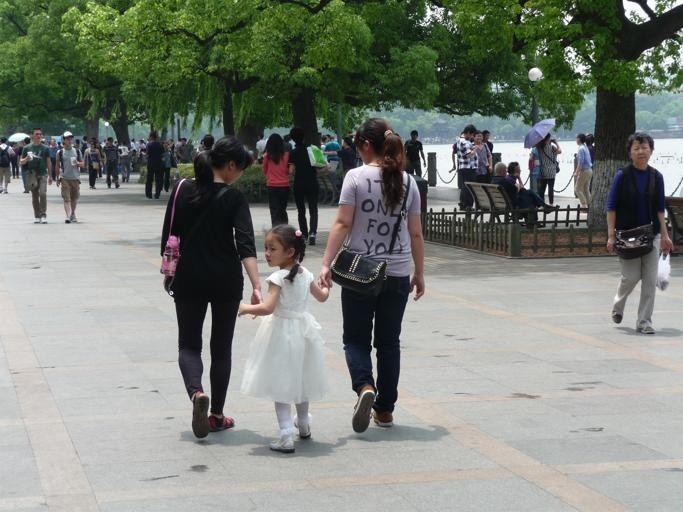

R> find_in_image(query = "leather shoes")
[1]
[544,206,560,214]
[526,222,544,229]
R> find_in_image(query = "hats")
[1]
[63,130,73,138]
[24,137,31,141]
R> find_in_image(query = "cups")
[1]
[27,151,33,161]
[70,156,76,166]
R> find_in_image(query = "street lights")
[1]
[529,66,542,126]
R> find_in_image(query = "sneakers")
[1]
[34,217,40,223]
[269,434,295,453]
[352,385,376,433]
[70,214,77,222]
[192,393,210,438]
[636,321,655,333]
[64,217,71,223]
[373,411,393,427]
[299,424,311,439]
[612,314,623,324]
[40,215,47,223]
[209,414,234,431]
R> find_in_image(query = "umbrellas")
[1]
[525,118,556,148]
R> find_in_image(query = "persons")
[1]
[605,132,674,334]
[318,122,424,434]
[585,134,596,194]
[160,138,214,192]
[146,132,162,199]
[288,127,318,245]
[573,132,592,211]
[76,136,146,188]
[472,131,492,184]
[529,142,540,193]
[482,130,493,150]
[238,225,329,452]
[508,162,524,192]
[263,134,290,227]
[318,133,360,171]
[0,128,83,224]
[458,124,475,211]
[491,162,560,228]
[537,132,561,210]
[404,130,426,177]
[160,136,262,438]
[452,130,465,154]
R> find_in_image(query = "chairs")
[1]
[662,196,683,247]
[463,180,538,224]
[315,168,343,208]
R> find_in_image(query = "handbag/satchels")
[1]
[331,173,411,297]
[89,147,100,169]
[542,147,559,173]
[306,143,327,168]
[24,145,45,191]
[615,166,656,260]
[170,151,177,169]
